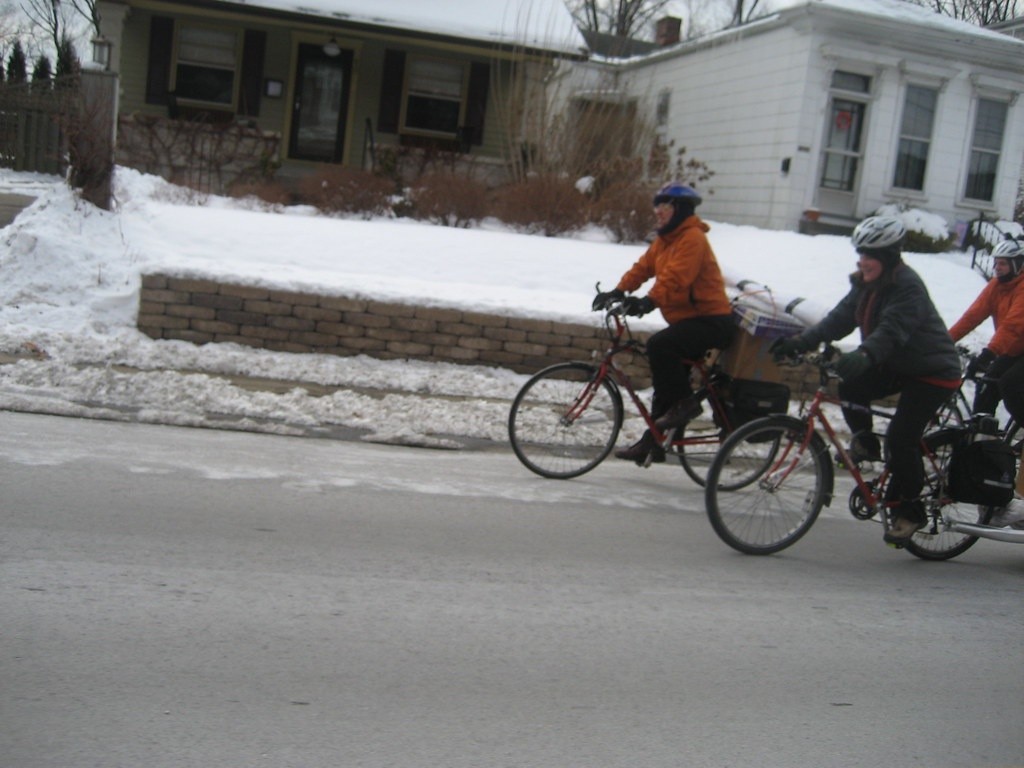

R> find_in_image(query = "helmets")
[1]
[851,215,906,249]
[991,237,1024,258]
[652,181,702,206]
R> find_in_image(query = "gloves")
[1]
[767,333,810,361]
[623,296,654,318]
[967,348,995,378]
[592,288,625,311]
[835,347,873,382]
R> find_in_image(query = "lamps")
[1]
[323,35,340,57]
[90,34,113,71]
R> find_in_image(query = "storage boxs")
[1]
[719,304,808,386]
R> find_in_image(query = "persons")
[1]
[604,179,734,463]
[949,240,1024,430]
[767,215,962,544]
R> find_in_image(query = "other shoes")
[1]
[883,515,928,544]
[835,448,881,463]
[654,396,703,429]
[614,430,666,462]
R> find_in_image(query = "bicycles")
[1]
[921,345,1024,532]
[507,280,790,492]
[704,335,1016,562]
